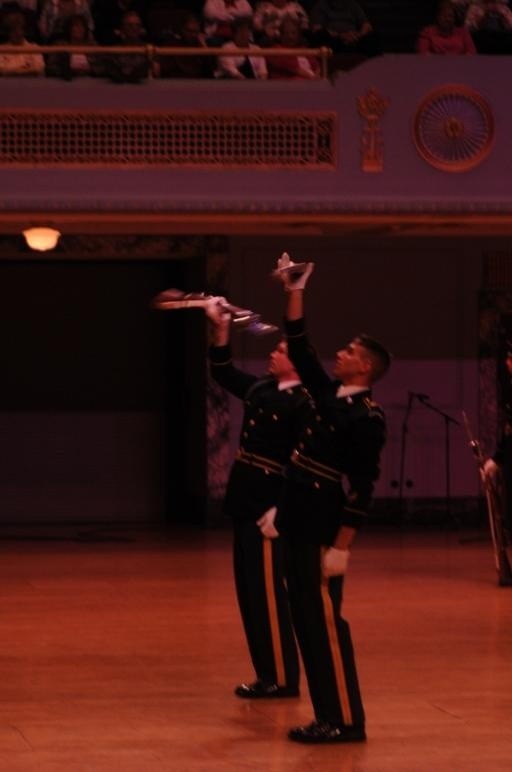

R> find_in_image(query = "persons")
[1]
[270,251,393,744]
[205,295,321,698]
[483,348,512,588]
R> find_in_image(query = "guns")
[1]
[461,410,509,573]
[153,287,278,334]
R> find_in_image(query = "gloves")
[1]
[255,505,279,539]
[321,547,351,578]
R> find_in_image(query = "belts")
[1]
[290,448,342,481]
[234,446,287,476]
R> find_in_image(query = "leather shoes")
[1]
[288,720,334,743]
[254,682,299,698]
[316,723,366,742]
[235,679,280,698]
